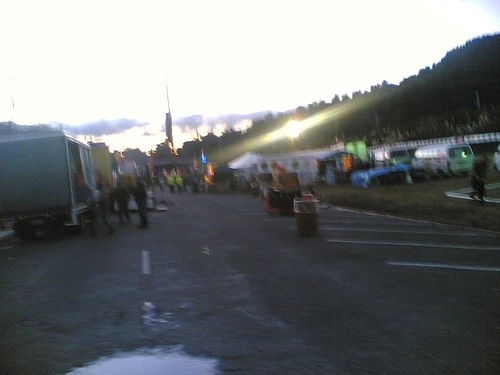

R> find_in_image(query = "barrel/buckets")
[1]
[258,172,301,214]
[293,199,320,237]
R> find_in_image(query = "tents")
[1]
[268,151,355,185]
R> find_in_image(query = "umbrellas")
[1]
[228,152,266,175]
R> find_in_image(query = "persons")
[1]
[469,150,486,204]
[105,179,131,225]
[85,183,115,237]
[129,178,147,229]
[157,169,207,193]
[493,145,500,172]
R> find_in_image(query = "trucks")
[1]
[0,131,100,238]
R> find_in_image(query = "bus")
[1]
[410,143,475,178]
